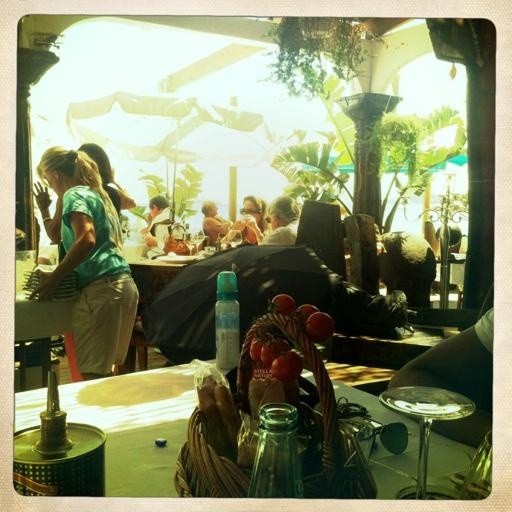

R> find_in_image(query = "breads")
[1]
[195,374,285,468]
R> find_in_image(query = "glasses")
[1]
[239,208,260,214]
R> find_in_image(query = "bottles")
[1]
[247,404,306,499]
[14,366,108,495]
[215,270,241,372]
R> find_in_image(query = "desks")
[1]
[14,246,491,499]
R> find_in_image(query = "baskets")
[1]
[171,312,380,497]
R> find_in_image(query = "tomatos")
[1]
[250,294,335,383]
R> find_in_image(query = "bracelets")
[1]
[257,235,263,241]
[42,217,52,225]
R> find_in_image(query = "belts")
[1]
[87,272,133,286]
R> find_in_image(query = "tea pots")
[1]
[163,226,199,257]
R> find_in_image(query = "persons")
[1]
[141,193,301,254]
[386,304,493,450]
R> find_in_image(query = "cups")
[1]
[463,430,492,501]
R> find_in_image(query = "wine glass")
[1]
[183,231,243,256]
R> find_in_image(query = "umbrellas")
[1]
[142,241,338,364]
[64,88,280,226]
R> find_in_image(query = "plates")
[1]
[24,267,81,299]
[157,253,205,262]
